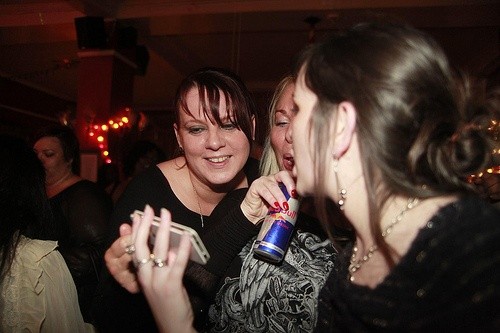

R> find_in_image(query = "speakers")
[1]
[75,16,108,50]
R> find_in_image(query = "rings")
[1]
[126,247,138,255]
[153,260,168,268]
[136,255,153,264]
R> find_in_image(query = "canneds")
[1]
[253,184,301,263]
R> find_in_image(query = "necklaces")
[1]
[189,173,207,227]
[349,197,420,283]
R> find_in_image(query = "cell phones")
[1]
[130,210,211,264]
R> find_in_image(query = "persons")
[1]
[0,15,499,333]
